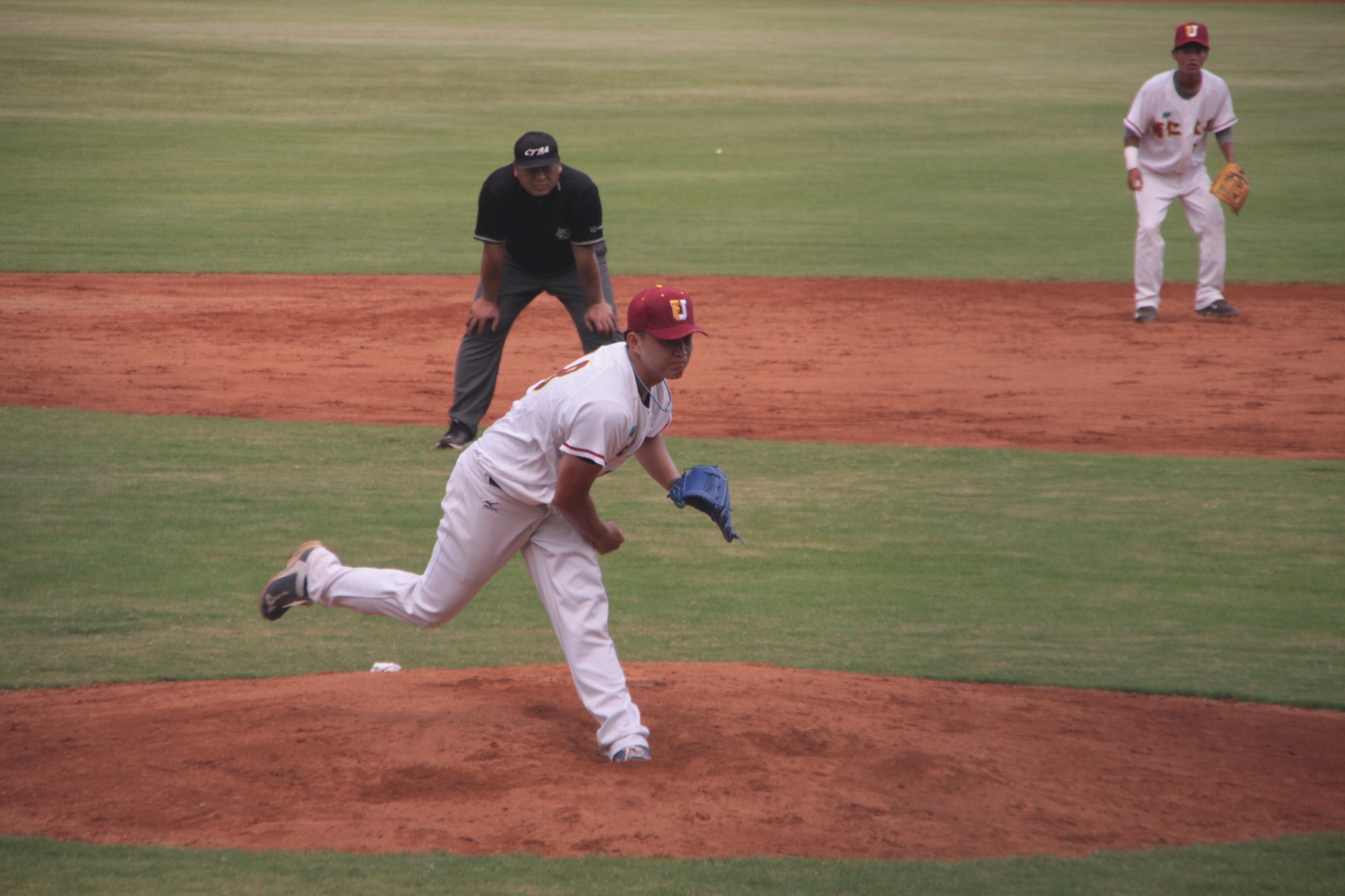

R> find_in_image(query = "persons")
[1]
[1124,22,1250,323]
[431,134,623,450]
[251,287,738,761]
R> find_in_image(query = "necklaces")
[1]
[626,350,672,413]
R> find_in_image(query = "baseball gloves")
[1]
[668,463,736,543]
[1210,163,1252,216]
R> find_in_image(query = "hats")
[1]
[627,284,710,340]
[1175,22,1209,48]
[514,131,560,168]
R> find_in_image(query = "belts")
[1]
[489,476,502,489]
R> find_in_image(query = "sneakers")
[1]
[1197,300,1239,316]
[259,541,324,622]
[436,424,476,450]
[613,745,650,763]
[1135,306,1156,321]
